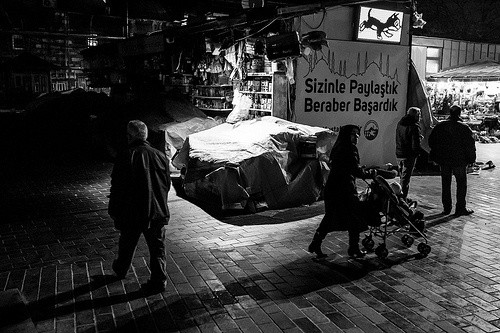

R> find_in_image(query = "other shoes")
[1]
[147,278,166,293]
[455,209,474,217]
[443,207,451,215]
[111,258,127,279]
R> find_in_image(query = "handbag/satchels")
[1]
[360,187,382,227]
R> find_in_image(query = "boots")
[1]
[347,243,366,258]
[308,231,328,257]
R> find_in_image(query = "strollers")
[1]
[362,164,431,258]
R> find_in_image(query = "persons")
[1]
[428,106,475,216]
[108,119,171,286]
[307,125,420,259]
[395,107,425,203]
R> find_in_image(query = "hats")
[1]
[127,120,148,141]
